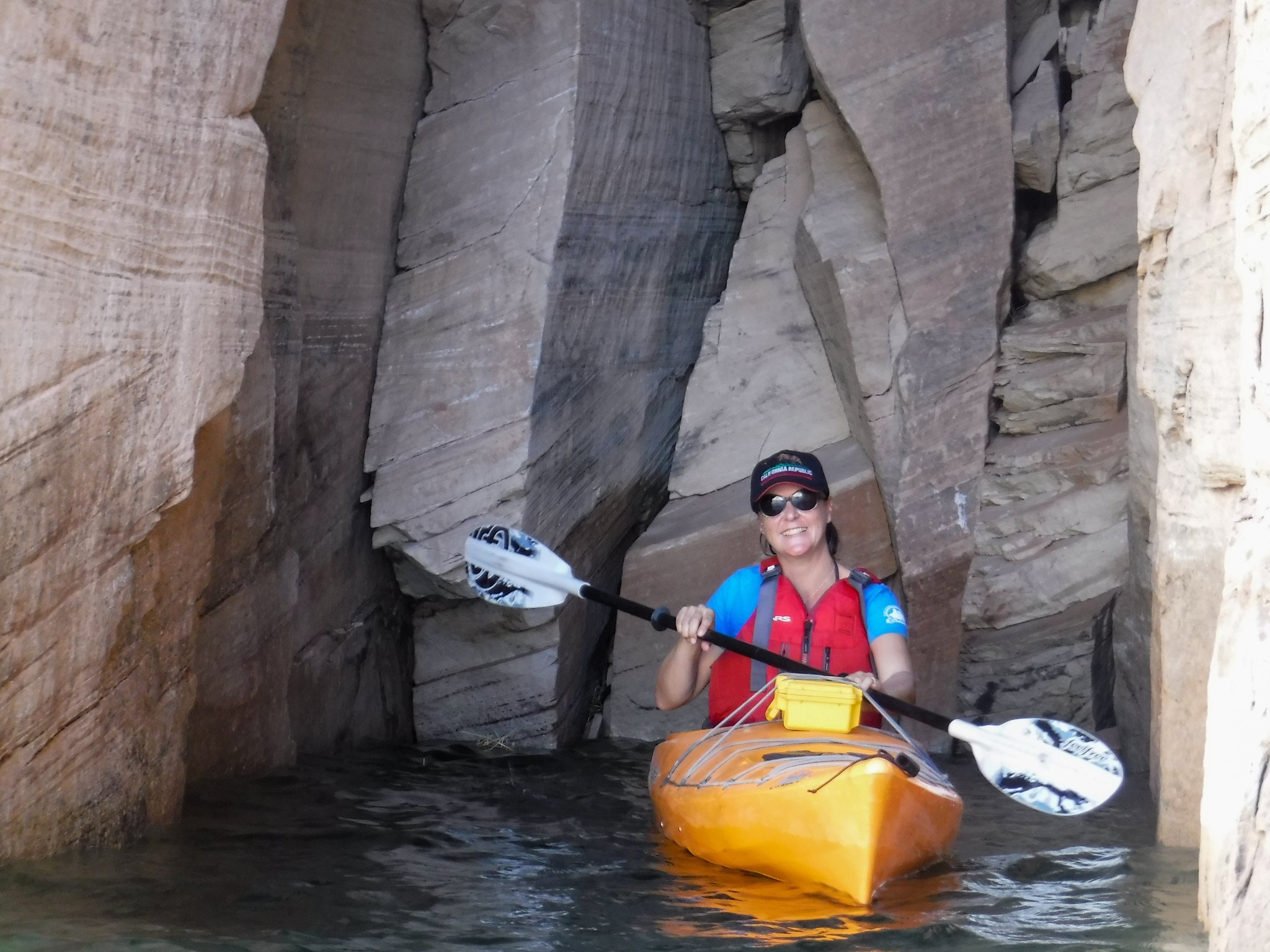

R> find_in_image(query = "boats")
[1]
[647,721,966,903]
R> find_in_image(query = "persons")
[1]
[656,451,917,729]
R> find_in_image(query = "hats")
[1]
[750,449,829,514]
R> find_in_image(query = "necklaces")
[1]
[803,564,835,604]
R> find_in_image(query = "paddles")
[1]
[464,522,1127,817]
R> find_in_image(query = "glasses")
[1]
[756,488,827,517]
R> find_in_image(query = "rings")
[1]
[871,677,876,684]
[868,672,873,676]
